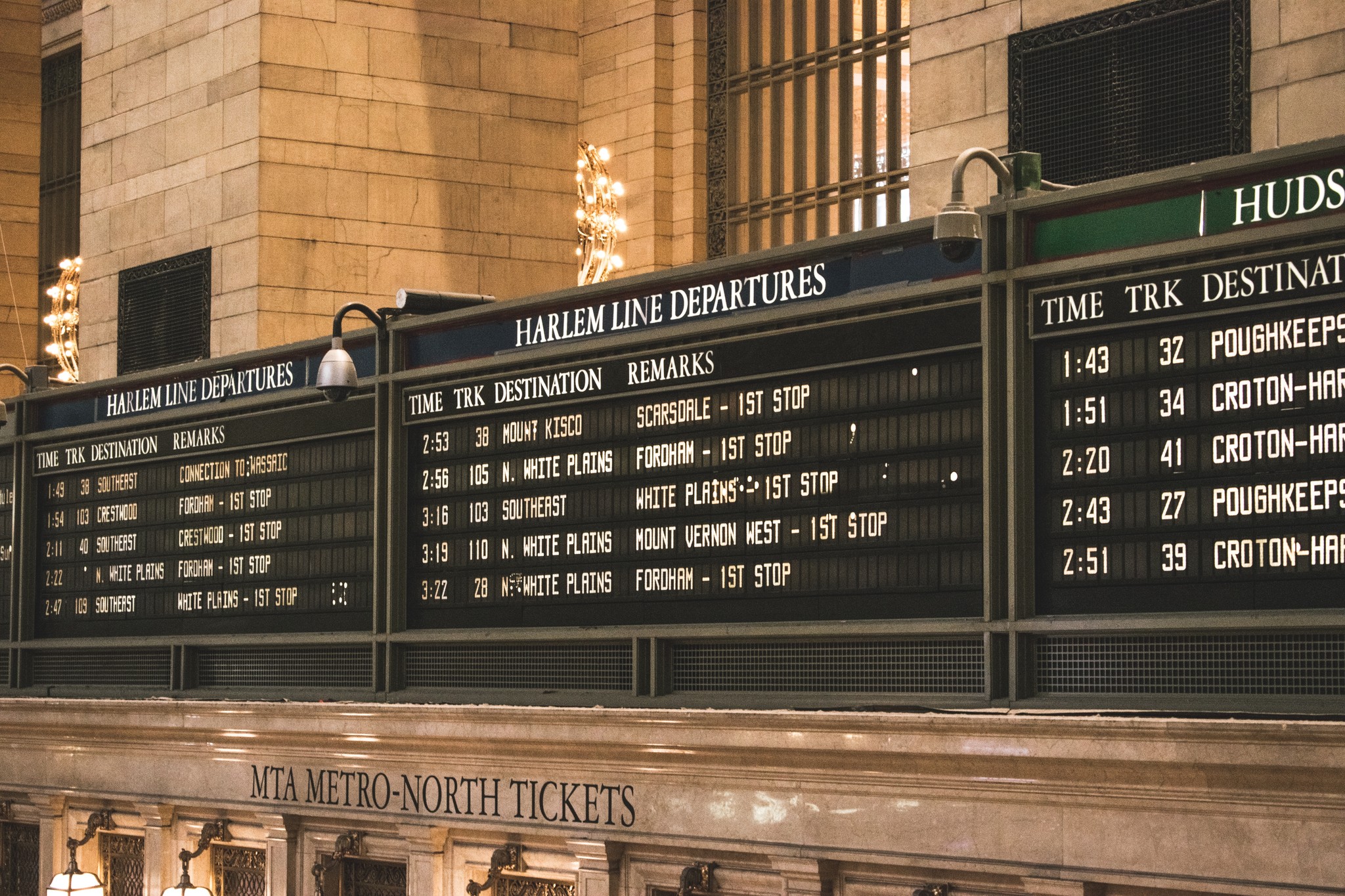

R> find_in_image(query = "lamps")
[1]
[159,821,223,896]
[311,830,363,896]
[0,364,28,430]
[46,809,118,896]
[466,843,523,896]
[315,302,386,405]
[932,147,1014,264]
